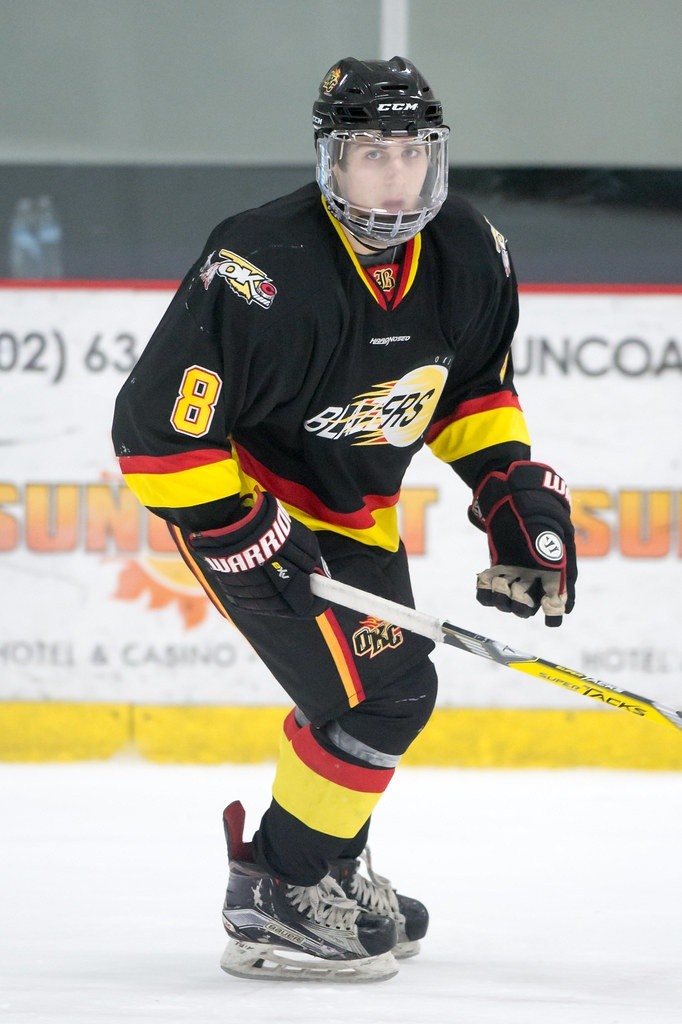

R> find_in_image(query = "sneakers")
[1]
[327,844,429,956]
[220,800,400,982]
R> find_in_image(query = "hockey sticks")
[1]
[305,569,682,739]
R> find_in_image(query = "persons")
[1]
[111,57,578,984]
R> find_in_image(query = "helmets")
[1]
[313,55,443,138]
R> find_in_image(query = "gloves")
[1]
[186,485,335,621]
[467,460,578,629]
